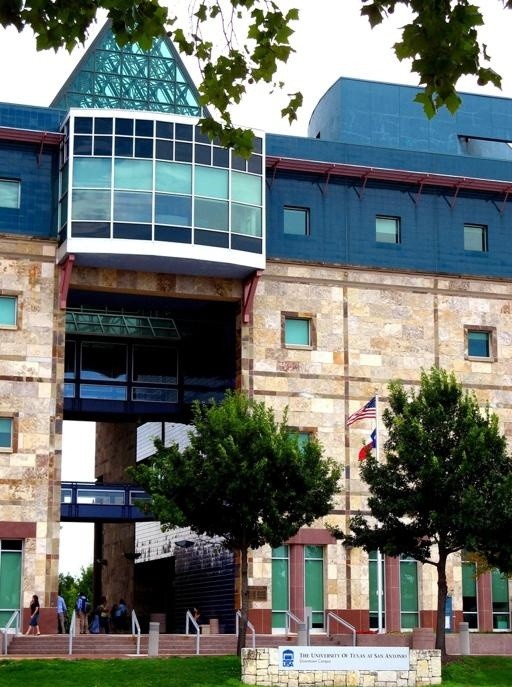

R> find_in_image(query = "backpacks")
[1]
[80,598,93,614]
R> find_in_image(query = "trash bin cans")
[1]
[412,628,436,650]
[210,619,219,634]
[151,613,167,634]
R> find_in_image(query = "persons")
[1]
[192,606,200,632]
[58,594,68,633]
[76,591,128,633]
[24,594,41,635]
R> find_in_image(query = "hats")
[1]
[78,592,85,596]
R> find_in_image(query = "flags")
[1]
[357,428,376,460]
[344,395,377,428]
